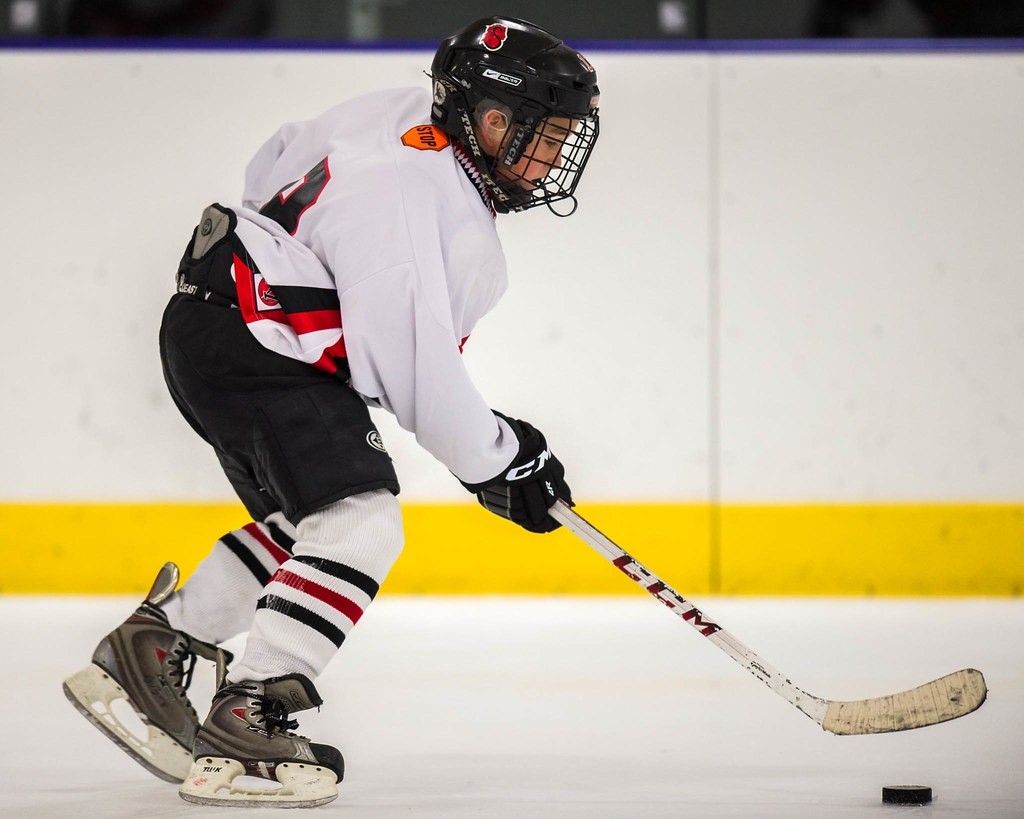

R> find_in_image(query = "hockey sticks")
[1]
[547,497,991,739]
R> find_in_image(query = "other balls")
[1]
[883,783,934,806]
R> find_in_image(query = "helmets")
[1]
[431,14,600,145]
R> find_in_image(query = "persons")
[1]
[60,13,603,810]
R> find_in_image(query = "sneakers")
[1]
[180,648,345,808]
[72,561,233,784]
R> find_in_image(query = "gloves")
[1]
[448,409,575,534]
